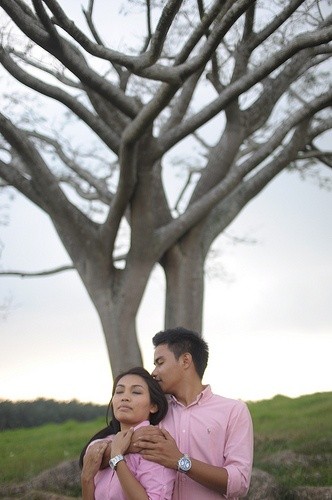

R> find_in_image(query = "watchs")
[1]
[176,454,192,474]
[109,455,127,470]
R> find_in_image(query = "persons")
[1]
[79,366,176,500]
[84,326,254,500]
